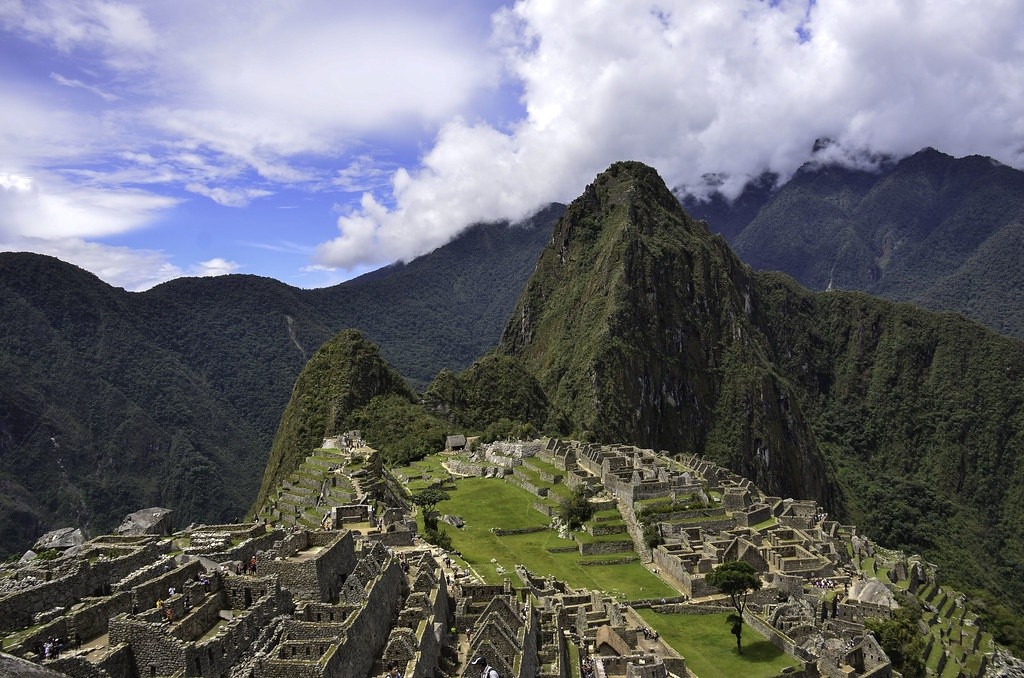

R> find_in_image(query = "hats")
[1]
[472,657,486,666]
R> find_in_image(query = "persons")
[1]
[812,578,837,590]
[33,476,659,678]
